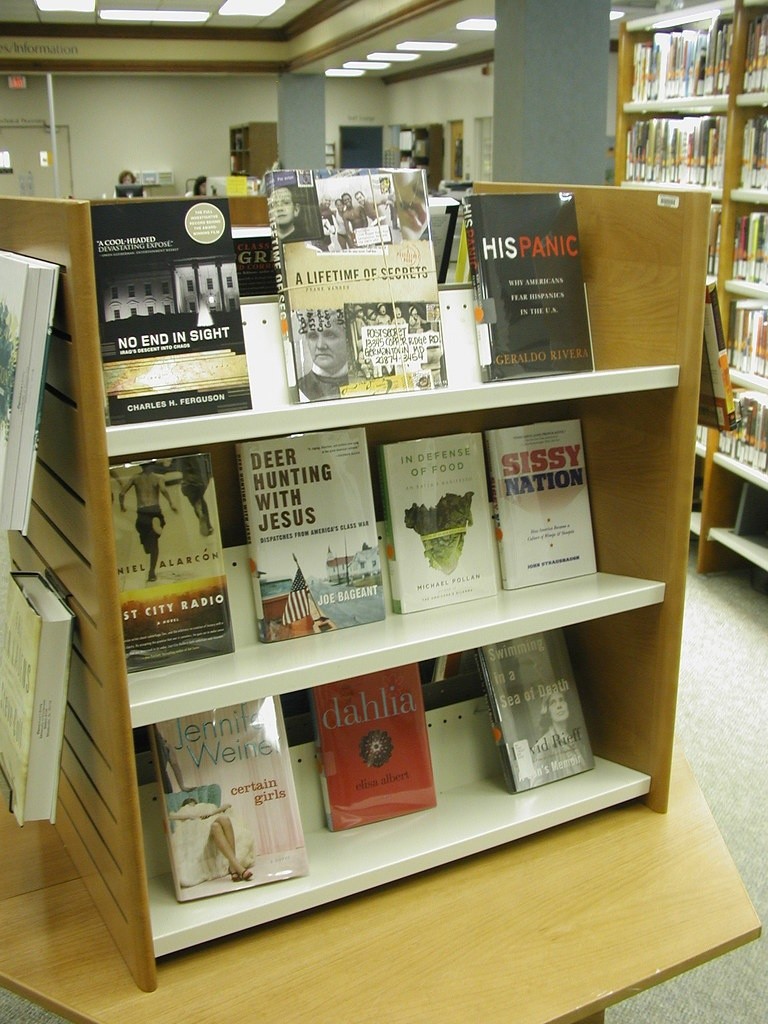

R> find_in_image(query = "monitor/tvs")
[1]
[116,185,143,198]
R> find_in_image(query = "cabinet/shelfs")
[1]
[0,0,768,1024]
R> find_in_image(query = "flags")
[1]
[281,569,311,626]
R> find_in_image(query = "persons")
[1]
[268,185,308,240]
[296,312,349,403]
[184,175,206,197]
[311,190,393,253]
[168,798,254,886]
[349,302,440,377]
[152,722,196,792]
[118,171,136,185]
[170,456,214,537]
[528,684,589,787]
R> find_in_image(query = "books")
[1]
[90,176,279,426]
[0,250,74,827]
[116,461,180,581]
[427,195,597,382]
[631,15,768,473]
[91,419,598,903]
